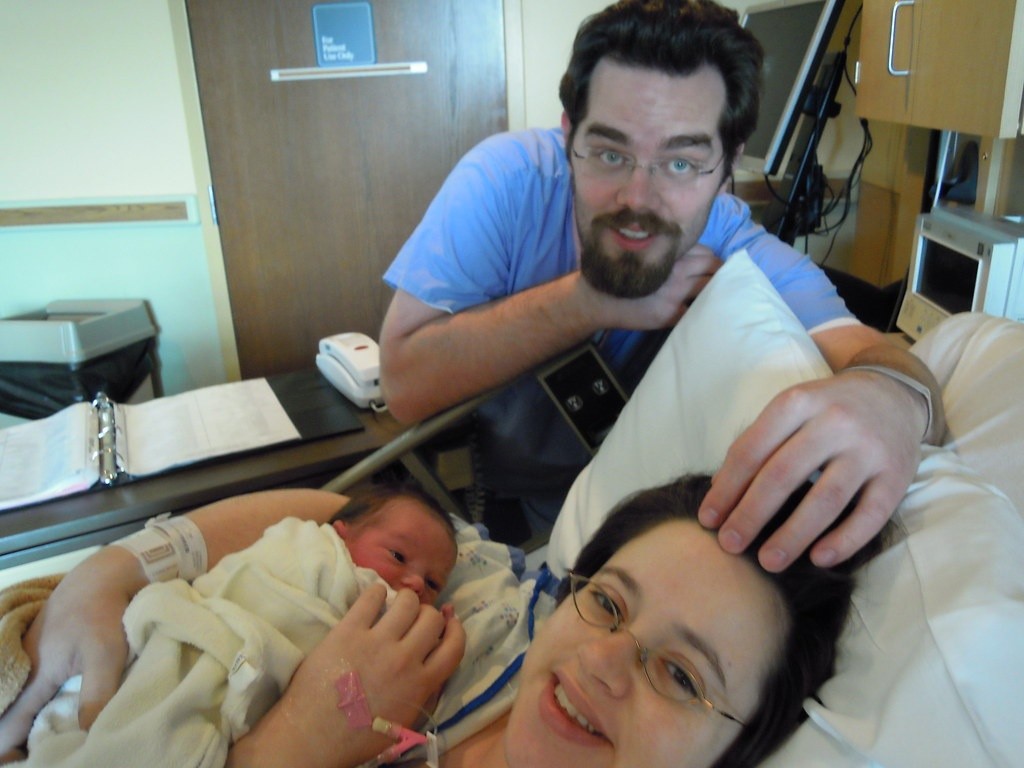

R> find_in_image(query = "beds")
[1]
[0,308,1024,768]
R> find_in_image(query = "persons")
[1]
[380,0,952,574]
[1,473,900,768]
[26,474,462,768]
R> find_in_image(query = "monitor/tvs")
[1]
[734,0,846,174]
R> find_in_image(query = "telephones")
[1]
[313,329,383,409]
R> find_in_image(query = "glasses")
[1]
[570,133,726,192]
[567,570,749,728]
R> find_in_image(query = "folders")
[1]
[0,362,365,513]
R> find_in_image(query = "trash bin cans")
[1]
[1,299,164,429]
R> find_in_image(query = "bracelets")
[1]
[841,364,933,442]
[110,511,209,583]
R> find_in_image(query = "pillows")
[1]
[544,248,1023,768]
